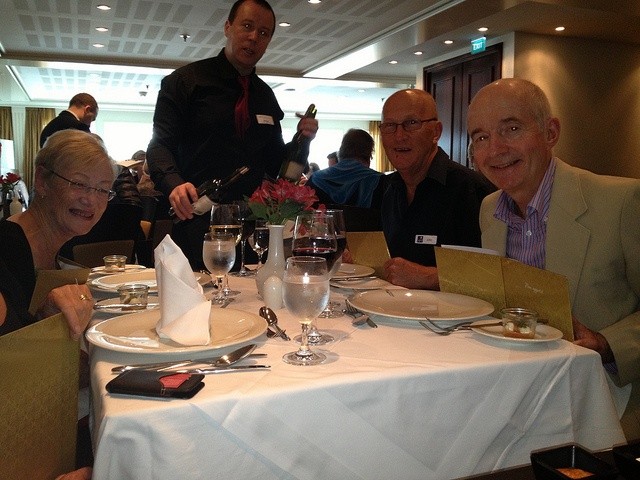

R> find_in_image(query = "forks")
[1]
[419,321,473,339]
[424,314,476,329]
[343,301,379,329]
[266,325,288,340]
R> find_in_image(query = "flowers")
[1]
[0,172,21,206]
[246,175,318,226]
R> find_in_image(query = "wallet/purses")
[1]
[104,368,205,398]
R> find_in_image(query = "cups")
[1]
[237,216,256,277]
[307,209,348,319]
[209,204,243,297]
[502,307,540,339]
[248,219,270,274]
[203,232,236,306]
[117,284,149,311]
[291,215,337,346]
[282,256,330,367]
[103,255,127,274]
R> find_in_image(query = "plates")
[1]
[91,271,201,292]
[344,287,499,324]
[469,319,564,342]
[85,307,269,355]
[93,295,161,314]
[331,262,376,279]
[89,264,146,275]
[329,277,389,288]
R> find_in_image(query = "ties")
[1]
[233,76,252,145]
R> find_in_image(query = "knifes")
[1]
[108,363,275,375]
[461,319,549,328]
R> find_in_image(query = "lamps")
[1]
[179,33,190,44]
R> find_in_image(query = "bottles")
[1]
[275,104,318,184]
[167,166,249,226]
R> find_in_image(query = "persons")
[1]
[40,92,115,260]
[341,89,495,292]
[146,0,318,273]
[1,129,118,343]
[305,128,388,232]
[327,152,338,166]
[467,77,639,442]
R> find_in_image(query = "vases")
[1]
[9,196,22,216]
[254,225,289,302]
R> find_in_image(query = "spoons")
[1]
[110,344,256,374]
[341,308,369,326]
[259,305,292,342]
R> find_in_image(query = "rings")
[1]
[80,294,89,301]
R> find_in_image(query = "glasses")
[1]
[378,117,438,135]
[48,168,117,202]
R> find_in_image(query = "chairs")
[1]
[73,240,135,269]
[152,220,172,266]
